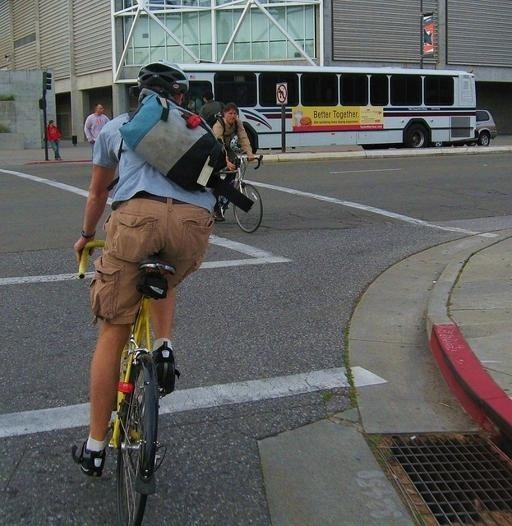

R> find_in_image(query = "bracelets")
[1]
[81,229,98,239]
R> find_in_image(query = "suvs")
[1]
[432,109,497,147]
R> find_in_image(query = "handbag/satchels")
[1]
[118,87,228,192]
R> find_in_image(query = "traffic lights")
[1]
[43,70,52,90]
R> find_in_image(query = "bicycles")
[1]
[72,239,177,526]
[211,153,263,234]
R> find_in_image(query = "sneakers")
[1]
[71,438,106,477]
[214,207,225,221]
[152,341,176,396]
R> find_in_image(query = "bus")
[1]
[129,56,477,151]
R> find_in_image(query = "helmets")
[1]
[136,59,190,94]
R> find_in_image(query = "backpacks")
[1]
[205,111,238,136]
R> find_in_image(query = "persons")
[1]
[46,119,64,162]
[69,58,218,479]
[82,102,112,161]
[168,89,256,224]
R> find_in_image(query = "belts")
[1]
[136,188,185,204]
